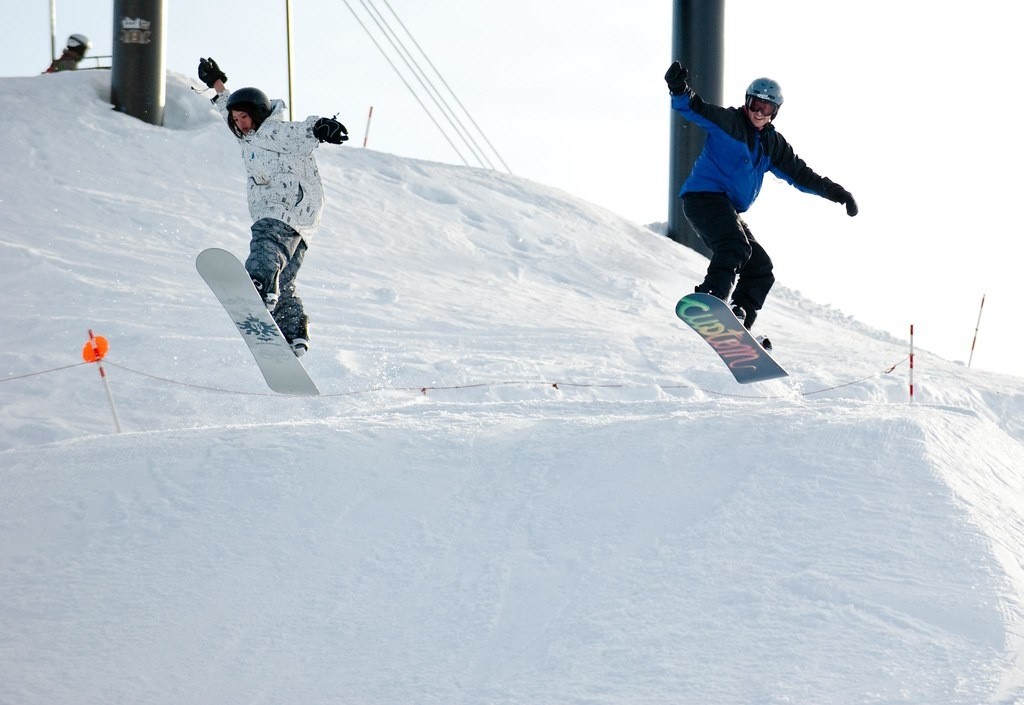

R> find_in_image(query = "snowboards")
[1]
[194,248,319,398]
[676,293,787,386]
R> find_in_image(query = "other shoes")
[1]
[251,269,282,312]
[728,299,773,353]
[282,315,311,357]
[695,284,747,320]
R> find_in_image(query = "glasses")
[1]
[747,95,778,116]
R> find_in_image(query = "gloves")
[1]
[664,61,688,96]
[313,112,348,144]
[191,57,228,94]
[826,183,858,217]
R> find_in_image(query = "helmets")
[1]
[226,87,272,111]
[745,77,784,106]
[67,34,92,48]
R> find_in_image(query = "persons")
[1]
[48,33,91,74]
[196,57,348,358]
[664,61,858,352]
